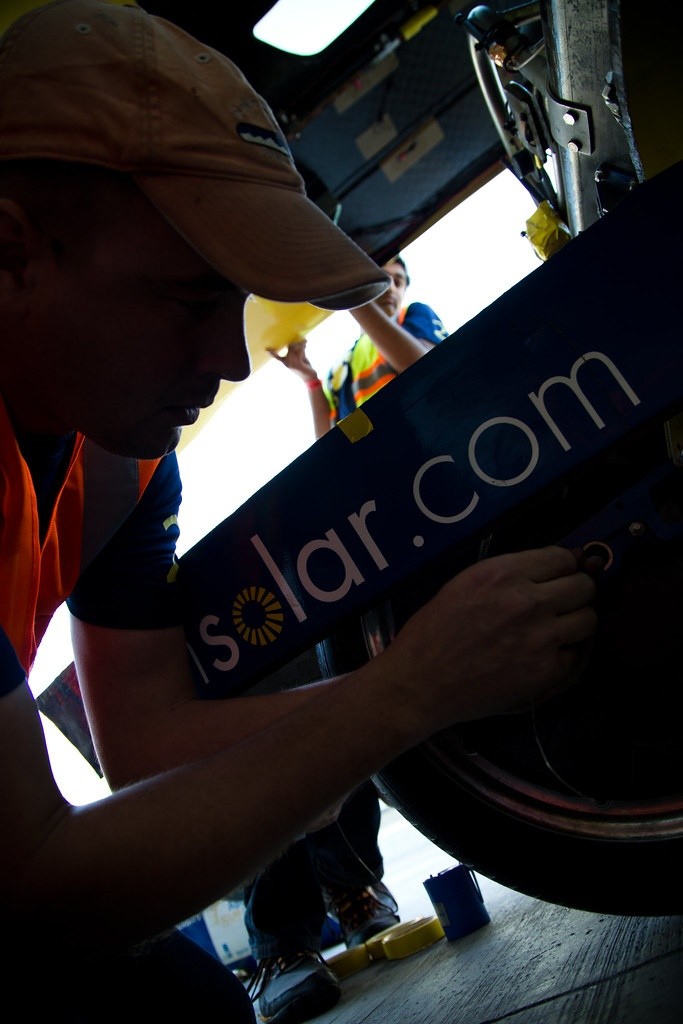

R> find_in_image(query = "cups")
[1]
[422,864,491,943]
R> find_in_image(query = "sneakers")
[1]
[246,951,340,1024]
[328,881,397,948]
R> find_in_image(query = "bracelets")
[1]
[306,379,322,389]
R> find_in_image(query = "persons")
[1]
[245,797,400,1024]
[0,1,595,1024]
[268,256,449,438]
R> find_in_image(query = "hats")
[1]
[0,0,391,311]
